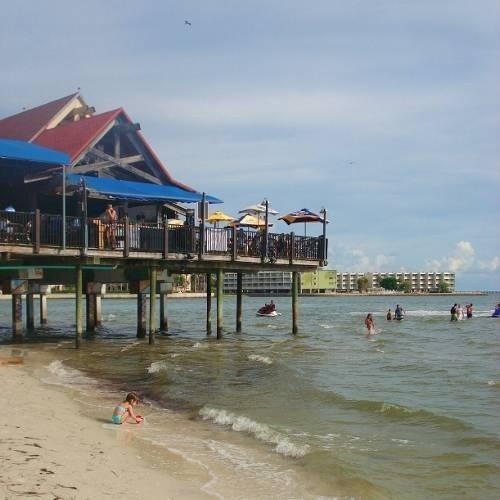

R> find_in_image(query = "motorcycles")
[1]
[255,307,278,318]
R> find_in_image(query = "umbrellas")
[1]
[277,208,329,236]
[204,210,236,228]
[238,202,279,227]
[231,214,273,231]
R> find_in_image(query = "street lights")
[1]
[78,174,90,250]
[199,192,210,259]
[260,198,271,259]
[320,206,328,261]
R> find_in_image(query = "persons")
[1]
[1,217,13,241]
[104,203,119,251]
[387,309,392,320]
[395,304,404,321]
[120,211,145,223]
[25,219,32,242]
[262,304,270,314]
[230,230,290,257]
[109,392,145,424]
[270,300,275,311]
[448,302,473,323]
[365,313,374,333]
[492,303,500,317]
